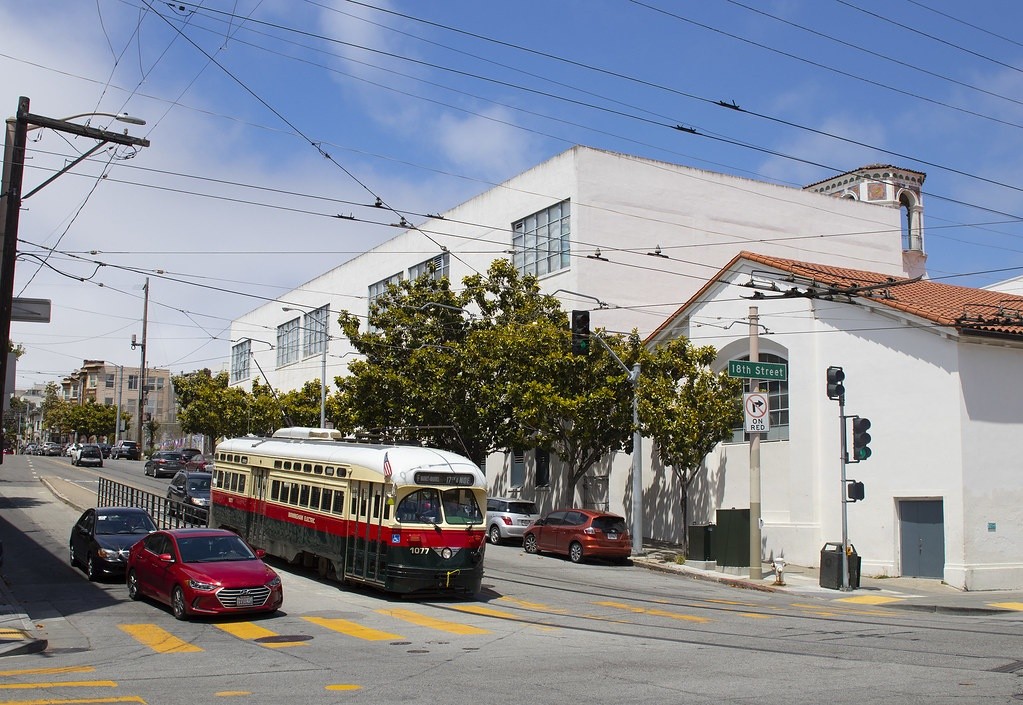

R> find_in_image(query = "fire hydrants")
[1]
[770,558,787,586]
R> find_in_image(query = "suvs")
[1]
[172,448,201,464]
[109,440,137,460]
[164,469,212,520]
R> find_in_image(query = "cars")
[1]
[69,507,169,581]
[62,442,103,467]
[126,528,283,621]
[26,442,62,456]
[523,508,632,564]
[144,451,186,478]
[97,443,112,459]
[186,454,214,474]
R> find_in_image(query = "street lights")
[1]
[58,376,80,407]
[0,111,147,273]
[282,307,327,429]
[94,358,124,446]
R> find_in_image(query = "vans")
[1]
[486,497,542,545]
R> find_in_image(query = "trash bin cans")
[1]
[819,543,861,590]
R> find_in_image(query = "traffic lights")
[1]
[853,417,872,460]
[827,366,845,400]
[572,309,590,356]
[848,482,865,501]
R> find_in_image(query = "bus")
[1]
[208,426,487,597]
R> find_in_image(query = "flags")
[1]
[384,452,392,477]
